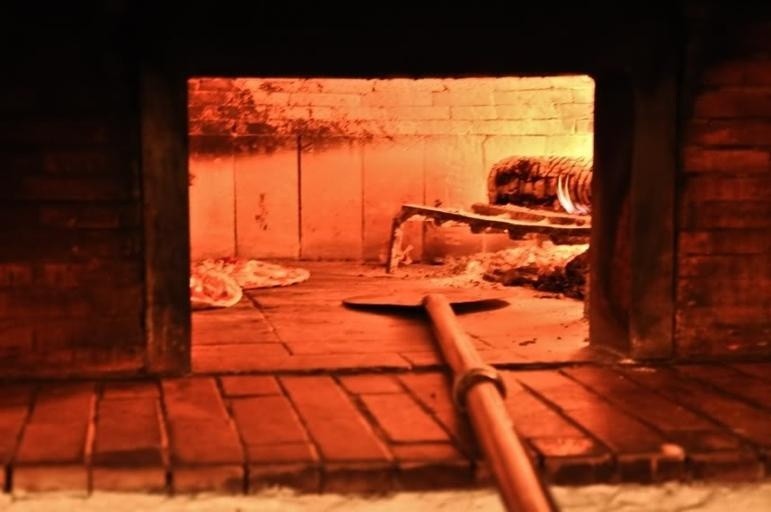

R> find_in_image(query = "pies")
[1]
[189,256,308,308]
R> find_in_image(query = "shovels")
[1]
[343,289,556,512]
